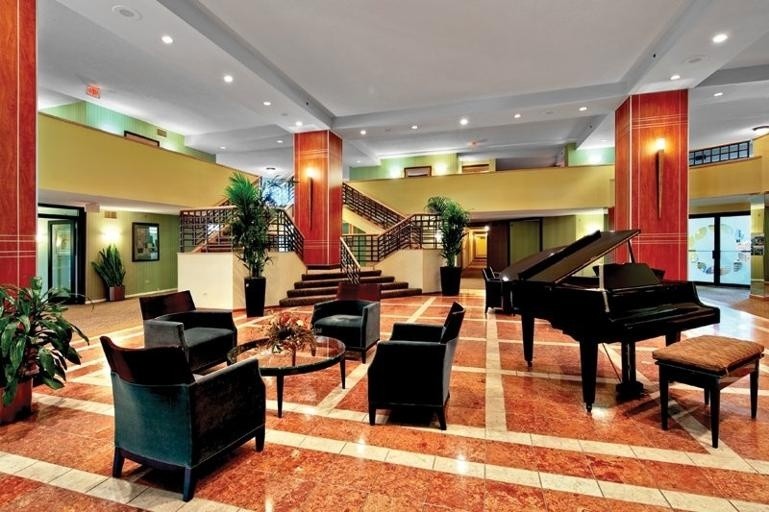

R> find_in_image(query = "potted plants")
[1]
[425,195,471,296]
[224,170,294,318]
[0,277,94,425]
[91,245,126,302]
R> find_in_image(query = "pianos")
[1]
[499,230,721,412]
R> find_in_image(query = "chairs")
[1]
[99,336,266,502]
[138,290,237,373]
[482,267,516,316]
[365,302,465,430]
[310,281,382,363]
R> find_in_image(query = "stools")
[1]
[652,336,765,450]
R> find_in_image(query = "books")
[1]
[336,314,361,320]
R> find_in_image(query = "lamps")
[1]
[655,138,666,219]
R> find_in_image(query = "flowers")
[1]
[256,308,321,356]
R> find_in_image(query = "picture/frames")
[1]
[132,222,160,262]
